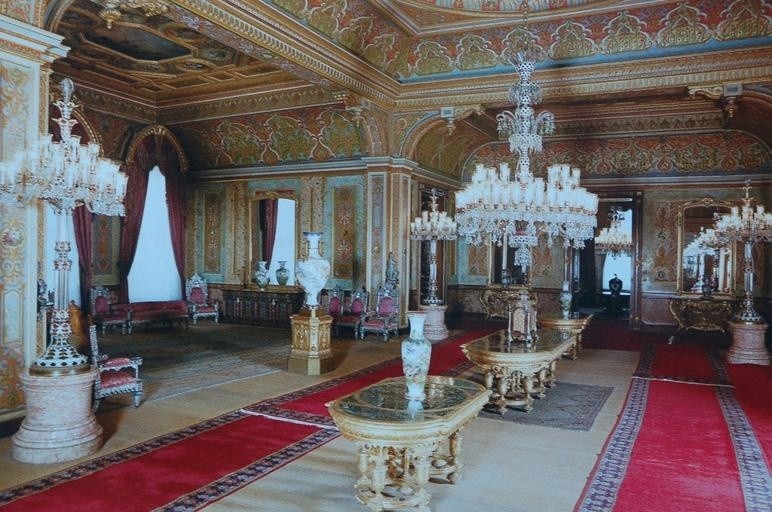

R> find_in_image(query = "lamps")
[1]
[683,227,715,291]
[409,186,458,306]
[706,213,734,294]
[712,176,772,323]
[1,76,130,378]
[595,205,634,261]
[455,62,599,274]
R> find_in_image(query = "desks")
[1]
[459,327,578,418]
[538,311,594,359]
[323,373,493,512]
[596,291,631,315]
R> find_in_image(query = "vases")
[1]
[254,261,271,287]
[608,273,623,297]
[295,231,331,306]
[275,260,290,285]
[560,280,572,320]
[400,310,432,396]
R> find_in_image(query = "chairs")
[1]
[88,323,143,414]
[360,279,401,343]
[185,272,220,325]
[90,280,127,339]
[336,285,368,339]
[325,284,345,337]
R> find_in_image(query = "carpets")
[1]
[578,314,742,355]
[1,323,515,510]
[574,341,771,512]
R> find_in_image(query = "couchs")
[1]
[128,301,188,334]
